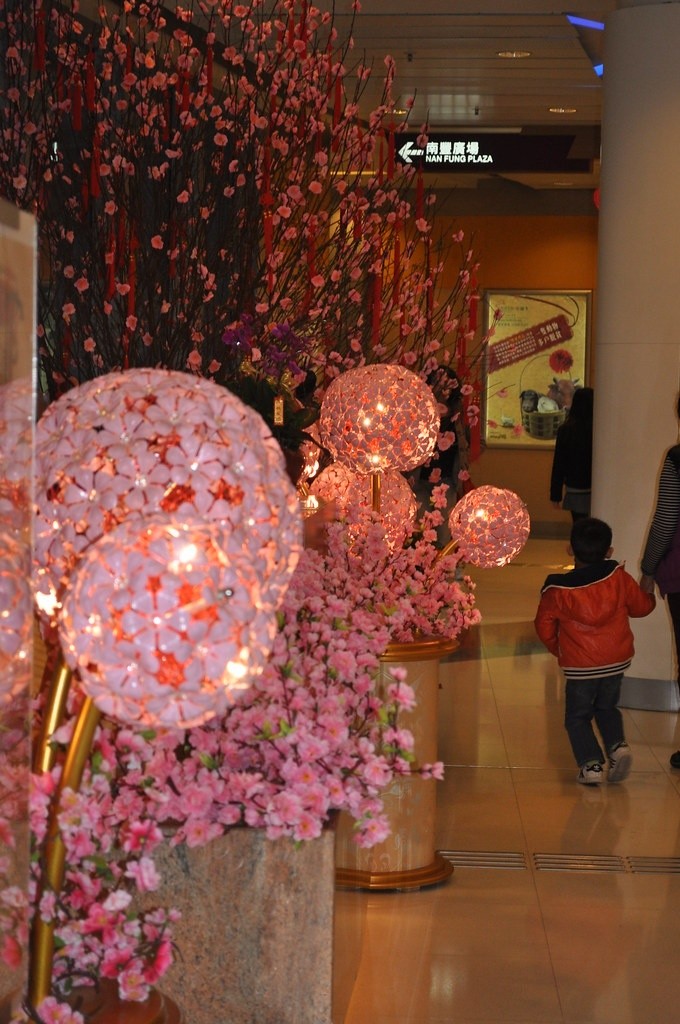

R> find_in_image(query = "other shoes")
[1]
[669,751,680,769]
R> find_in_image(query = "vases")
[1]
[102,802,340,1024]
[0,977,184,1024]
[333,634,455,892]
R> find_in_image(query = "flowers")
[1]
[0,0,502,1024]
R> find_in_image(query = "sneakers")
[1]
[577,760,603,783]
[607,742,633,781]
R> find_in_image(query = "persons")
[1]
[549,387,594,554]
[237,364,470,579]
[640,394,680,767]
[533,518,657,784]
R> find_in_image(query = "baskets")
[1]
[519,355,573,440]
